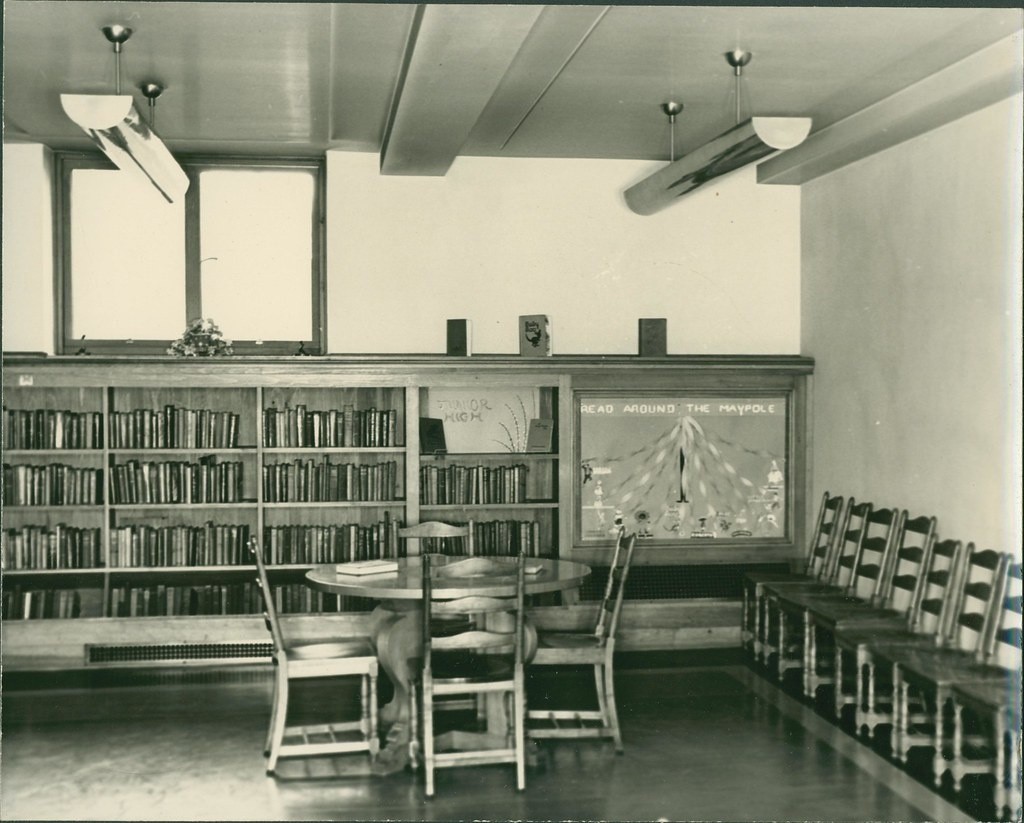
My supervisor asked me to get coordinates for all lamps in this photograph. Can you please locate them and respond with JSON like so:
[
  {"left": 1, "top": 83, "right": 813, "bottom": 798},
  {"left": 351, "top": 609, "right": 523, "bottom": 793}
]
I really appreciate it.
[
  {"left": 623, "top": 52, "right": 812, "bottom": 220},
  {"left": 58, "top": 24, "right": 193, "bottom": 206}
]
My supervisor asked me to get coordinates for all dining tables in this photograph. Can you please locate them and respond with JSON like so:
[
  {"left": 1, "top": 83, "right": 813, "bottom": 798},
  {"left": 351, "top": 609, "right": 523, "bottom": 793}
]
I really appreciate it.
[{"left": 303, "top": 553, "right": 594, "bottom": 770}]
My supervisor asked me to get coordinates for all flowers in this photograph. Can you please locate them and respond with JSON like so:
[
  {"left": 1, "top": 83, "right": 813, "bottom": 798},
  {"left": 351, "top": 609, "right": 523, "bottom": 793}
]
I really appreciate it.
[{"left": 166, "top": 318, "right": 235, "bottom": 359}]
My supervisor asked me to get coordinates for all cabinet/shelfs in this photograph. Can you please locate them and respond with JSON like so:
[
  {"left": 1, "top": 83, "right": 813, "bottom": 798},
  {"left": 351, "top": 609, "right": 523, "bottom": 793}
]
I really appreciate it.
[{"left": 0, "top": 354, "right": 582, "bottom": 688}]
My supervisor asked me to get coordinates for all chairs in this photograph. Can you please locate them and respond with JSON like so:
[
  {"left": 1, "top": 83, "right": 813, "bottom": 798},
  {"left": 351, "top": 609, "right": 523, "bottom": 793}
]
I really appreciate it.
[
  {"left": 396, "top": 516, "right": 487, "bottom": 726},
  {"left": 508, "top": 525, "right": 639, "bottom": 754},
  {"left": 741, "top": 489, "right": 1024, "bottom": 822},
  {"left": 244, "top": 532, "right": 382, "bottom": 780},
  {"left": 406, "top": 548, "right": 528, "bottom": 799}
]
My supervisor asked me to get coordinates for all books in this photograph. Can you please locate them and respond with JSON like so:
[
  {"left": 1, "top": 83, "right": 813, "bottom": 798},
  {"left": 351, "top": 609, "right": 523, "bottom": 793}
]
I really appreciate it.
[{"left": 1, "top": 402, "right": 543, "bottom": 613}]
